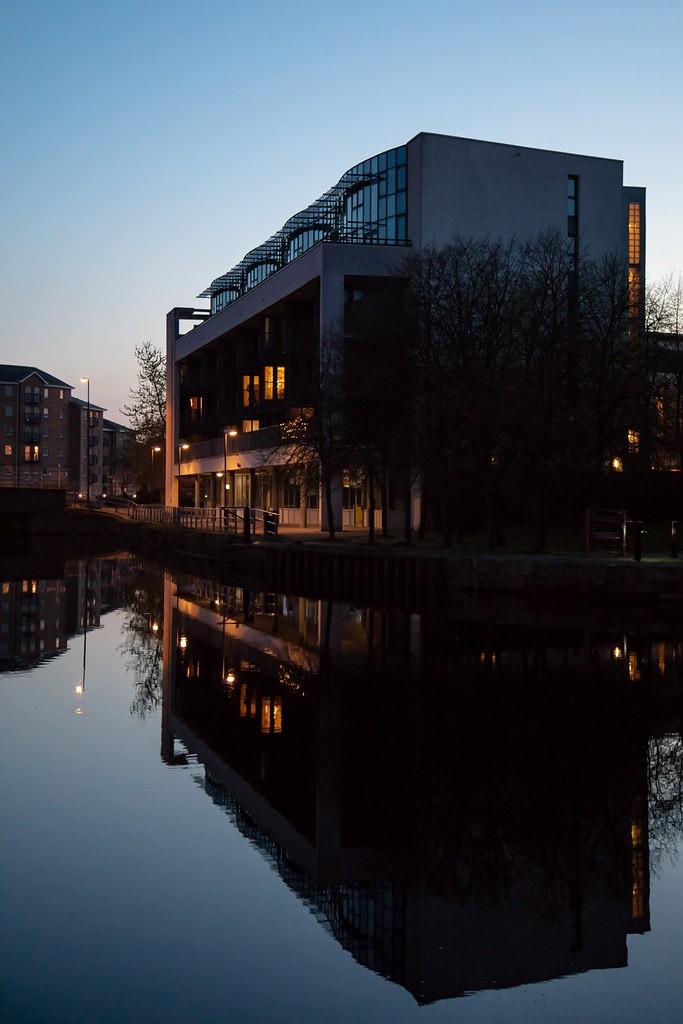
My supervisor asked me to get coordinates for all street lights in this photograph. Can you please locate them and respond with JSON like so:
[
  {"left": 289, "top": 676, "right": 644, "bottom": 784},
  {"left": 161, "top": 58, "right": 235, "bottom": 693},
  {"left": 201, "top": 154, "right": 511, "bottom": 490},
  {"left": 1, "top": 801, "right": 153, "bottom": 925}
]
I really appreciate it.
[
  {"left": 223, "top": 427, "right": 238, "bottom": 533},
  {"left": 220, "top": 605, "right": 235, "bottom": 683},
  {"left": 81, "top": 375, "right": 91, "bottom": 510},
  {"left": 73, "top": 560, "right": 89, "bottom": 695},
  {"left": 149, "top": 444, "right": 163, "bottom": 504},
  {"left": 177, "top": 442, "right": 191, "bottom": 509}
]
